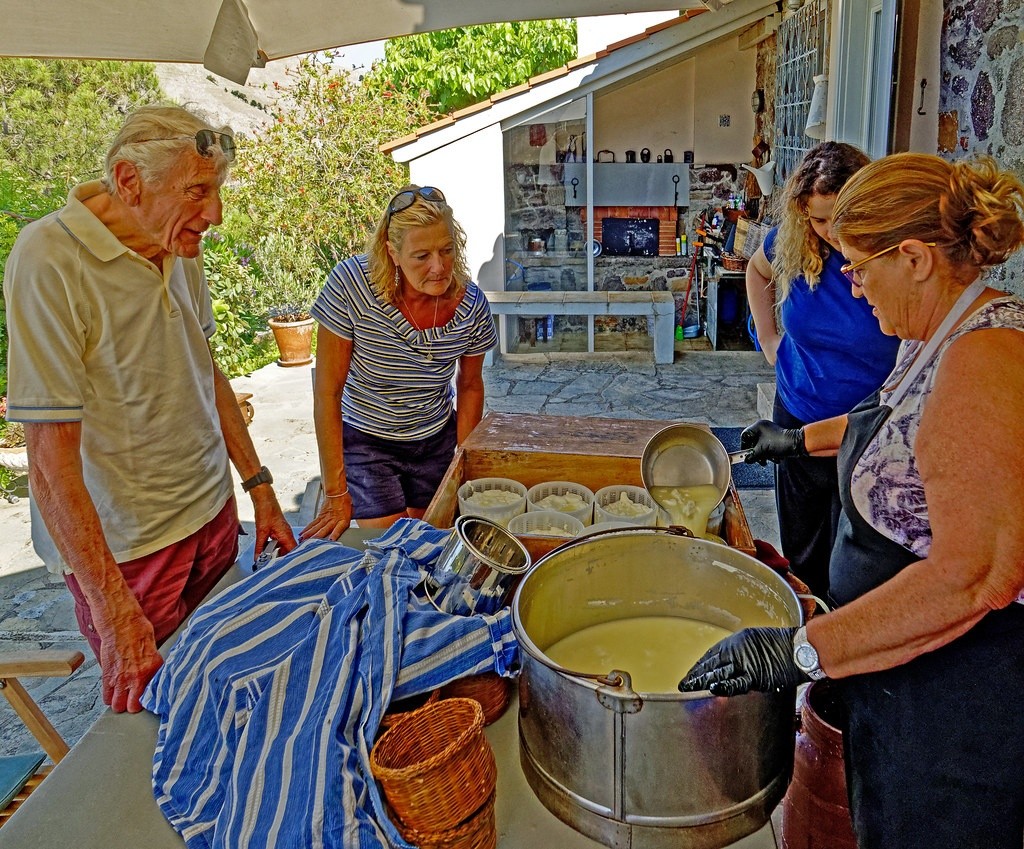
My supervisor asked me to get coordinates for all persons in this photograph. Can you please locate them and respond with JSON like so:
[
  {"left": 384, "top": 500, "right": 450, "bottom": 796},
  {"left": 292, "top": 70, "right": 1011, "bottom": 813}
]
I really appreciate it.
[
  {"left": 3, "top": 101, "right": 297, "bottom": 715},
  {"left": 746, "top": 139, "right": 900, "bottom": 607},
  {"left": 678, "top": 150, "right": 1023, "bottom": 849},
  {"left": 296, "top": 183, "right": 498, "bottom": 542}
]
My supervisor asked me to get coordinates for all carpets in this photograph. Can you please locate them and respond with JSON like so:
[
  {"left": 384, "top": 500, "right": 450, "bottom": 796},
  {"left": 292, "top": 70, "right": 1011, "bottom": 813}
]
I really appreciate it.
[{"left": 709, "top": 426, "right": 777, "bottom": 490}]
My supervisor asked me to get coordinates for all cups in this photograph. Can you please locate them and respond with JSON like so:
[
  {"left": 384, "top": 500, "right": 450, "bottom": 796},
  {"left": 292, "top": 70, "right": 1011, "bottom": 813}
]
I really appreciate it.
[{"left": 530, "top": 238, "right": 542, "bottom": 255}]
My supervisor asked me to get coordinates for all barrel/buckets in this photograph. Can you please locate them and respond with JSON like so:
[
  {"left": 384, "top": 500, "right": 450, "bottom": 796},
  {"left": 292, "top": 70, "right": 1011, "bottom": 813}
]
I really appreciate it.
[
  {"left": 510, "top": 525, "right": 802, "bottom": 849},
  {"left": 527, "top": 481, "right": 594, "bottom": 528},
  {"left": 595, "top": 485, "right": 657, "bottom": 534},
  {"left": 782, "top": 682, "right": 860, "bottom": 849},
  {"left": 657, "top": 502, "right": 728, "bottom": 547},
  {"left": 527, "top": 282, "right": 554, "bottom": 340},
  {"left": 508, "top": 511, "right": 584, "bottom": 537},
  {"left": 577, "top": 521, "right": 655, "bottom": 538},
  {"left": 457, "top": 478, "right": 527, "bottom": 530},
  {"left": 424, "top": 514, "right": 531, "bottom": 616}
]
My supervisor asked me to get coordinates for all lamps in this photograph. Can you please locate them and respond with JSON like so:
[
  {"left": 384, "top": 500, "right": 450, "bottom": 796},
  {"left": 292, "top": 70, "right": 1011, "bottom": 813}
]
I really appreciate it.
[{"left": 750, "top": 89, "right": 762, "bottom": 112}]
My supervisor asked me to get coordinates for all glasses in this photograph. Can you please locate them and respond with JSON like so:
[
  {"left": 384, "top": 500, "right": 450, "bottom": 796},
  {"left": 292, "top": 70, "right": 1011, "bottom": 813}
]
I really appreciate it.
[
  {"left": 134, "top": 129, "right": 237, "bottom": 163},
  {"left": 840, "top": 242, "right": 937, "bottom": 287},
  {"left": 385, "top": 187, "right": 447, "bottom": 230}
]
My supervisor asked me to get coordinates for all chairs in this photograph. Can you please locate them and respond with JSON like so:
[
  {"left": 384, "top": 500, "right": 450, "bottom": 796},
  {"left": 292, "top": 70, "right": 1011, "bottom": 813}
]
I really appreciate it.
[{"left": 0, "top": 650, "right": 85, "bottom": 829}]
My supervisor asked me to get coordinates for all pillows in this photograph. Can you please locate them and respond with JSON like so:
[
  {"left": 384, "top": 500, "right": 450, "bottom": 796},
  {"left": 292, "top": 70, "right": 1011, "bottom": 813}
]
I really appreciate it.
[{"left": 0, "top": 754, "right": 48, "bottom": 813}]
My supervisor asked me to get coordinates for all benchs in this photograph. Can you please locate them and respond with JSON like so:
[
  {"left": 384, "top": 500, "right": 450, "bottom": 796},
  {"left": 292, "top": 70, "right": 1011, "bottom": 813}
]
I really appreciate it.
[{"left": 482, "top": 291, "right": 674, "bottom": 367}]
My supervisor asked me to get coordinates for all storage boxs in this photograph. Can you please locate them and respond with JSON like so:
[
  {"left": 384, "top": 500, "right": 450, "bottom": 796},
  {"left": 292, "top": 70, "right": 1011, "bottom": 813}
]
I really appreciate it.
[{"left": 421, "top": 444, "right": 757, "bottom": 607}]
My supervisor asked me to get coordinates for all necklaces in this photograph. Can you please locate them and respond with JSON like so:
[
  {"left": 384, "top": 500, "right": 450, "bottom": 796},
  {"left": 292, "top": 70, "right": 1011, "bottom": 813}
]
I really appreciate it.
[{"left": 401, "top": 292, "right": 439, "bottom": 362}]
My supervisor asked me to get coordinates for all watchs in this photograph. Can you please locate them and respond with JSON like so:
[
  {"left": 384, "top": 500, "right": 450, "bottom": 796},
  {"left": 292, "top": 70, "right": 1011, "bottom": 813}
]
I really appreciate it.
[
  {"left": 792, "top": 625, "right": 828, "bottom": 682},
  {"left": 241, "top": 465, "right": 274, "bottom": 493}
]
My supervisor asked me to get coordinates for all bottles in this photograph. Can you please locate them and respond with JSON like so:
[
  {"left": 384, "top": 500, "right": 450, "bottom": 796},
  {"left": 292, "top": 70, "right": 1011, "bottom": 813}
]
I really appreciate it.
[
  {"left": 681, "top": 235, "right": 686, "bottom": 255},
  {"left": 722, "top": 194, "right": 745, "bottom": 210},
  {"left": 677, "top": 235, "right": 680, "bottom": 256}
]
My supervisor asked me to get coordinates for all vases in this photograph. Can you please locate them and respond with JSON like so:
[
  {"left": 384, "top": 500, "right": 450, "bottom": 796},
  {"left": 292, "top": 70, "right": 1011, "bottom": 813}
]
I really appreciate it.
[
  {"left": 267, "top": 317, "right": 316, "bottom": 367},
  {"left": 0, "top": 438, "right": 30, "bottom": 475}
]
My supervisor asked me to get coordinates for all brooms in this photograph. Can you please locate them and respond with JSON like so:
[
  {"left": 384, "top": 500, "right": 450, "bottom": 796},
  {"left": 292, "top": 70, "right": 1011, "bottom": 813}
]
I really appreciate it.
[{"left": 675, "top": 208, "right": 707, "bottom": 341}]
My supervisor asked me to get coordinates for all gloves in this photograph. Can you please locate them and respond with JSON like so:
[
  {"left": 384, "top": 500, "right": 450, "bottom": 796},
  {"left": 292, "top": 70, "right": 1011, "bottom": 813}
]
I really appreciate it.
[
  {"left": 677, "top": 626, "right": 814, "bottom": 697},
  {"left": 740, "top": 419, "right": 811, "bottom": 466}
]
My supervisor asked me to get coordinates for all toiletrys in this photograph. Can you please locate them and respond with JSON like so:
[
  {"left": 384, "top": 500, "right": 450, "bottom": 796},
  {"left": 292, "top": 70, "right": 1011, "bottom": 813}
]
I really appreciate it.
[{"left": 727, "top": 191, "right": 743, "bottom": 209}]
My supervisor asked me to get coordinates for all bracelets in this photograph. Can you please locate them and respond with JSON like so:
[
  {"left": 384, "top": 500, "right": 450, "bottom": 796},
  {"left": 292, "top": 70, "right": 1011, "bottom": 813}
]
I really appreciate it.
[{"left": 323, "top": 487, "right": 349, "bottom": 498}]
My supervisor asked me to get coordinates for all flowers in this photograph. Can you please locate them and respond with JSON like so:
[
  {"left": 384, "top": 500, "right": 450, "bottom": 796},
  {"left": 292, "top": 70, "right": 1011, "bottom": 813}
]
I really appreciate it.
[{"left": 0, "top": 396, "right": 28, "bottom": 449}]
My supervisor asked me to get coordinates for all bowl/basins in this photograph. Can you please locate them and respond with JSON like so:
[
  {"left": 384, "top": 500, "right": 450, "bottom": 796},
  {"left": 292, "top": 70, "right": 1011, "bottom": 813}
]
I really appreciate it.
[
  {"left": 722, "top": 207, "right": 750, "bottom": 223},
  {"left": 752, "top": 140, "right": 770, "bottom": 159}
]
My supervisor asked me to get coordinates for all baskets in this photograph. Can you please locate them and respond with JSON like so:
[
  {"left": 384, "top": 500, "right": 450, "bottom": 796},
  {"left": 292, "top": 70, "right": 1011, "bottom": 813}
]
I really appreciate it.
[
  {"left": 368, "top": 696, "right": 499, "bottom": 834},
  {"left": 385, "top": 784, "right": 497, "bottom": 848},
  {"left": 381, "top": 687, "right": 442, "bottom": 731},
  {"left": 442, "top": 672, "right": 510, "bottom": 729}
]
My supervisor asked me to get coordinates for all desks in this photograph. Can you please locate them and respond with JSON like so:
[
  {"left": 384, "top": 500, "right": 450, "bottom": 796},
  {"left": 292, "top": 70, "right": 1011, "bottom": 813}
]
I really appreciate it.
[
  {"left": 463, "top": 409, "right": 709, "bottom": 457},
  {"left": 0, "top": 523, "right": 775, "bottom": 849},
  {"left": 707, "top": 257, "right": 748, "bottom": 350}
]
